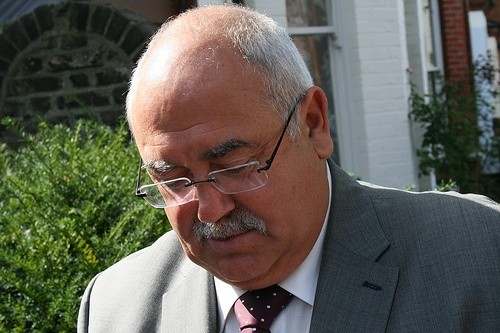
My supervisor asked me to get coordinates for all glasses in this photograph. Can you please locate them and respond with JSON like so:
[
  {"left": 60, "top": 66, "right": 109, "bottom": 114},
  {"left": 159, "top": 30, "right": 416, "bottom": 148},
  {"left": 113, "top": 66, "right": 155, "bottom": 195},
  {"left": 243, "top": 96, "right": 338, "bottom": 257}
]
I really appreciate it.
[{"left": 135, "top": 98, "right": 303, "bottom": 208}]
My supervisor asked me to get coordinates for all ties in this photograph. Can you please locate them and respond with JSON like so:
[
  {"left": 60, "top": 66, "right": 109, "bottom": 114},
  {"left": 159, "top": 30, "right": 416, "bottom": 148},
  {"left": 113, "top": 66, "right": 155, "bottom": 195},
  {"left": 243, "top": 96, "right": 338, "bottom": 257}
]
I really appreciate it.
[{"left": 232, "top": 285, "right": 296, "bottom": 333}]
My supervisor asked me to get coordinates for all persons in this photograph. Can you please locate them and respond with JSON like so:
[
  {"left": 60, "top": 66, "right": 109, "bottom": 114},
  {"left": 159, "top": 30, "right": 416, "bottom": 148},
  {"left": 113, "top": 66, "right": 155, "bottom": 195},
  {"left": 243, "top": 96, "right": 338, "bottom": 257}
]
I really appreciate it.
[{"left": 77, "top": 5, "right": 500, "bottom": 333}]
